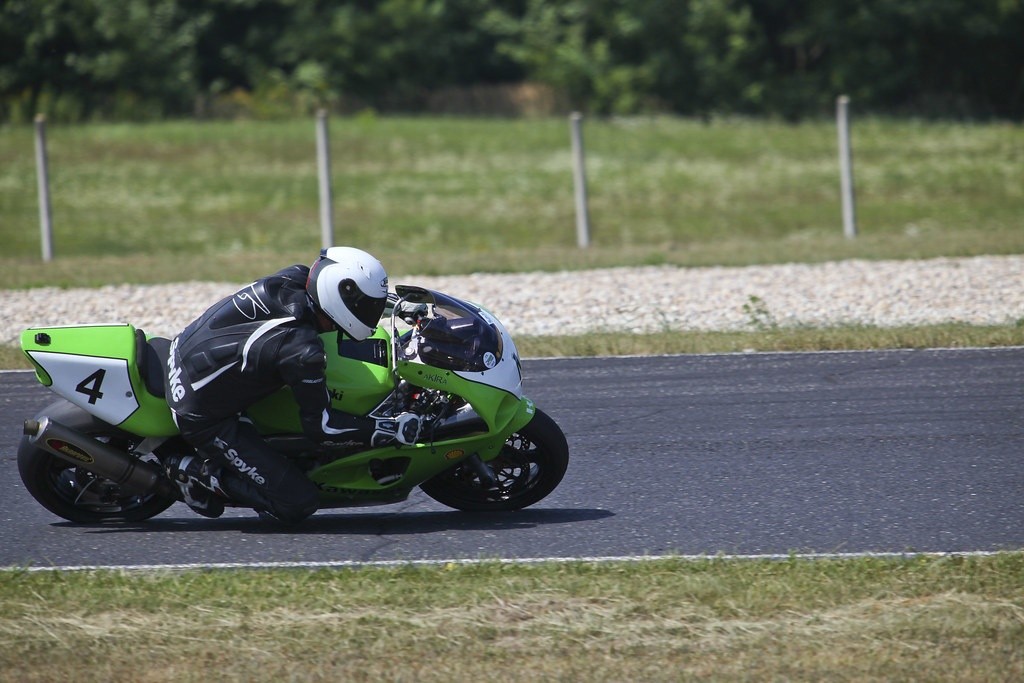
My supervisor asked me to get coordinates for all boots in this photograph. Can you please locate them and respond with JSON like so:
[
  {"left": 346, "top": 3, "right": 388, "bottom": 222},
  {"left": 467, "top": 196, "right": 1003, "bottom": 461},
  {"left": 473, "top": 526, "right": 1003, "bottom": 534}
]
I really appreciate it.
[{"left": 164, "top": 452, "right": 225, "bottom": 518}]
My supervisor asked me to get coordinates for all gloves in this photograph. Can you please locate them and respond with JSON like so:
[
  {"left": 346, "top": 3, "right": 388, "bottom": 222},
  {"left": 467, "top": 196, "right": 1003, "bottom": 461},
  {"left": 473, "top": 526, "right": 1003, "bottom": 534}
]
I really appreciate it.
[
  {"left": 380, "top": 292, "right": 429, "bottom": 325},
  {"left": 371, "top": 412, "right": 424, "bottom": 449}
]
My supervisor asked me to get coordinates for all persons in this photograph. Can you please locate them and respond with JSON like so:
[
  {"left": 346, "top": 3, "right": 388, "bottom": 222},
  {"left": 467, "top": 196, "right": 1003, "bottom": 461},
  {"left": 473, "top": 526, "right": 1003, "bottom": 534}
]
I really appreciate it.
[{"left": 165, "top": 247, "right": 435, "bottom": 528}]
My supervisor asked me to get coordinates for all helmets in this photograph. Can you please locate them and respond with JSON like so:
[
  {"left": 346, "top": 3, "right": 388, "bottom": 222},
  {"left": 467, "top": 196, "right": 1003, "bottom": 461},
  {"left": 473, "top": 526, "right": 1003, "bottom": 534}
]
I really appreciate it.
[{"left": 306, "top": 246, "right": 388, "bottom": 342}]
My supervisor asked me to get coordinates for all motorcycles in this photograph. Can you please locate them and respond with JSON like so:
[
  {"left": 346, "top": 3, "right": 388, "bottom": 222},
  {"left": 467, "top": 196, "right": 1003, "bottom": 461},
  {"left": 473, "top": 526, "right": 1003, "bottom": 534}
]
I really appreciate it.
[{"left": 13, "top": 281, "right": 571, "bottom": 528}]
